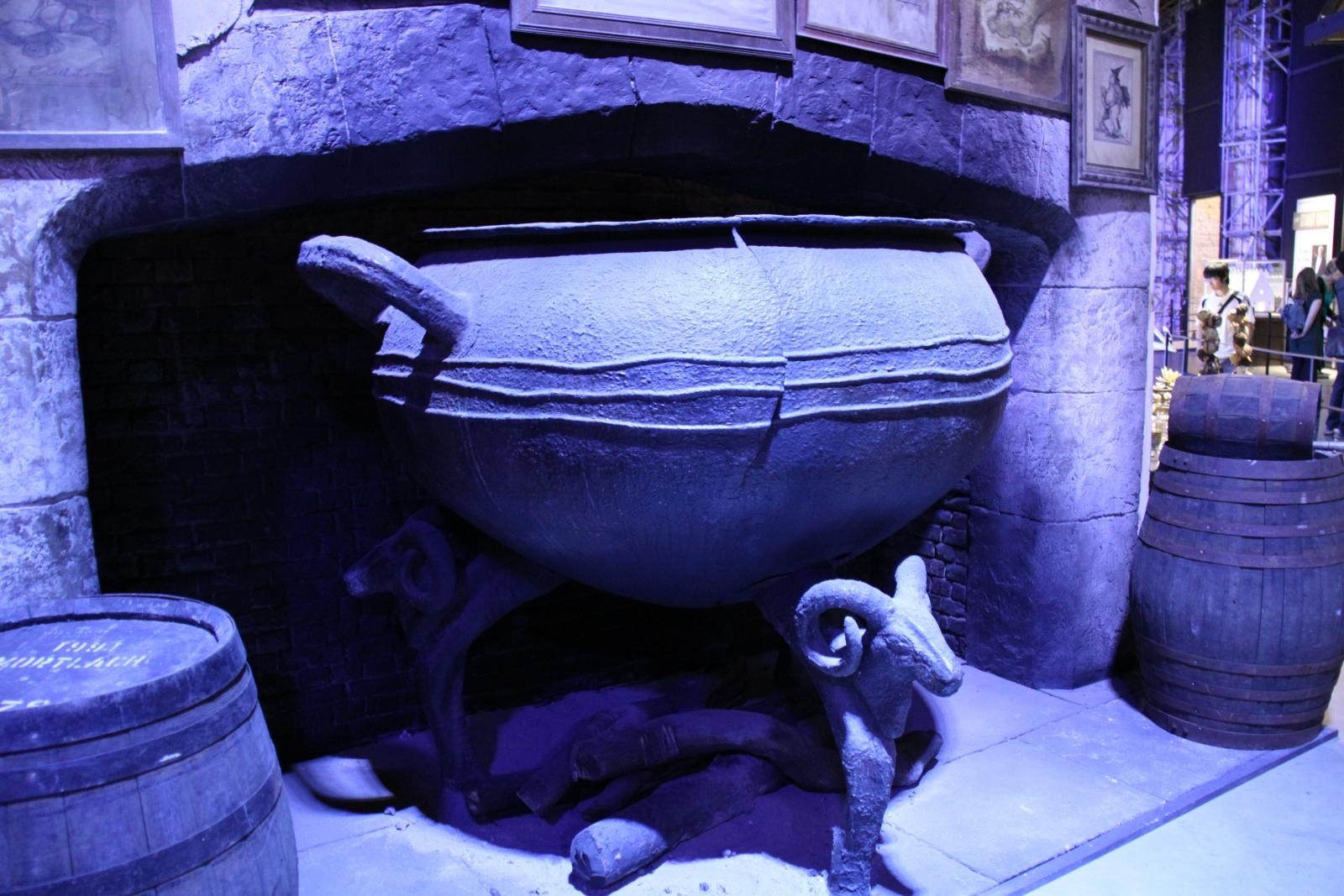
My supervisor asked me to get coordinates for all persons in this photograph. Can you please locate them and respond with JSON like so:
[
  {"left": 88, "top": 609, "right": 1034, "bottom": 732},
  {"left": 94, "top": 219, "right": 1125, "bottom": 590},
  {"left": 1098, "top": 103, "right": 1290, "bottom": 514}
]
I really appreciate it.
[
  {"left": 1289, "top": 268, "right": 1324, "bottom": 382},
  {"left": 1317, "top": 258, "right": 1338, "bottom": 378},
  {"left": 1324, "top": 251, "right": 1343, "bottom": 439},
  {"left": 1195, "top": 261, "right": 1256, "bottom": 376}
]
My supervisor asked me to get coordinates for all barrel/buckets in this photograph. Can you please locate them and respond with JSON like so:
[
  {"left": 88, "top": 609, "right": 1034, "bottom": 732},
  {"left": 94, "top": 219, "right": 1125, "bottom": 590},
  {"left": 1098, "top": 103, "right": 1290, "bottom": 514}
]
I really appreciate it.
[
  {"left": 1128, "top": 446, "right": 1340, "bottom": 749},
  {"left": 1167, "top": 374, "right": 1322, "bottom": 454},
  {"left": 0, "top": 590, "right": 303, "bottom": 896}
]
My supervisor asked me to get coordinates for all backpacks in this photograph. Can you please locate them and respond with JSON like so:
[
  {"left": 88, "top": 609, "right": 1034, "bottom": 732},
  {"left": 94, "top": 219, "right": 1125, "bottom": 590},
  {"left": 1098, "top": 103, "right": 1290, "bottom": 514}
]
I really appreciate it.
[{"left": 1282, "top": 296, "right": 1307, "bottom": 334}]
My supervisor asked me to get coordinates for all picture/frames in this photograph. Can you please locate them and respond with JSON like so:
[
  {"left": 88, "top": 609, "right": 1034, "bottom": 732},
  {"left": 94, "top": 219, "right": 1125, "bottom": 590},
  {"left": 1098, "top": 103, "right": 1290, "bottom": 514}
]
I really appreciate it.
[
  {"left": 945, "top": 1, "right": 1076, "bottom": 118},
  {"left": 1, "top": 0, "right": 184, "bottom": 156},
  {"left": 507, "top": 0, "right": 799, "bottom": 68},
  {"left": 1066, "top": 4, "right": 1160, "bottom": 197},
  {"left": 790, "top": 0, "right": 945, "bottom": 75}
]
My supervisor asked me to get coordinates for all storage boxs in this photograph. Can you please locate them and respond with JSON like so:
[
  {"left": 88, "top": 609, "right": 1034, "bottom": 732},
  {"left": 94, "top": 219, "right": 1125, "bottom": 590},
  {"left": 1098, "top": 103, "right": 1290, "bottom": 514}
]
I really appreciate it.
[{"left": 1219, "top": 257, "right": 1286, "bottom": 314}]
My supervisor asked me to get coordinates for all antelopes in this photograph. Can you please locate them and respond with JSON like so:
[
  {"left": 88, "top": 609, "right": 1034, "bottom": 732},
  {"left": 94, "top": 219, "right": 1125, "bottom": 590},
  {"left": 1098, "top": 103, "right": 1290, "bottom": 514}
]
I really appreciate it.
[{"left": 792, "top": 554, "right": 964, "bottom": 738}]
